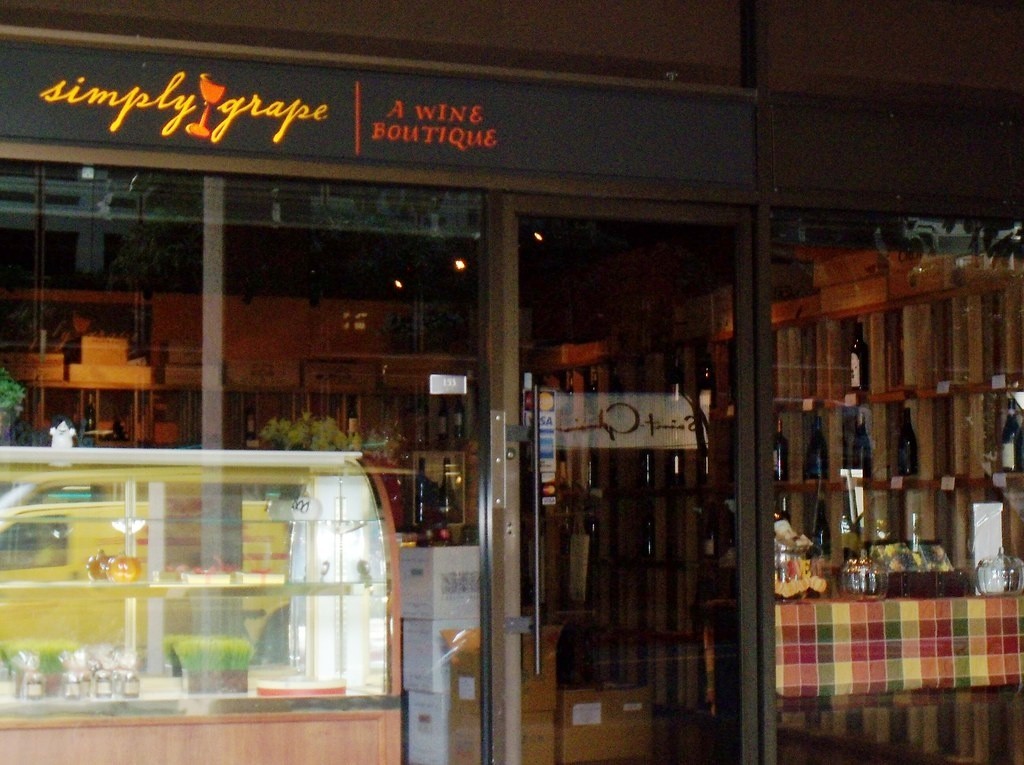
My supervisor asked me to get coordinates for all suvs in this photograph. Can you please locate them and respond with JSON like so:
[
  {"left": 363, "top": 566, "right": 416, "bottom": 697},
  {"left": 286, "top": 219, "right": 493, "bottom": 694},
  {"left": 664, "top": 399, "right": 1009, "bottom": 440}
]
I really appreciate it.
[
  {"left": 0, "top": 502, "right": 295, "bottom": 663},
  {"left": 0, "top": 456, "right": 399, "bottom": 678}
]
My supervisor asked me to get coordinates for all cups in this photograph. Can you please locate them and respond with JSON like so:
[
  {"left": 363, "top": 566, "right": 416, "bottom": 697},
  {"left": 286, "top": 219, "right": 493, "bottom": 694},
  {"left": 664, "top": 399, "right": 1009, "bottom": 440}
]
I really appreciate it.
[
  {"left": 976, "top": 547, "right": 1024, "bottom": 596},
  {"left": 836, "top": 549, "right": 889, "bottom": 601}
]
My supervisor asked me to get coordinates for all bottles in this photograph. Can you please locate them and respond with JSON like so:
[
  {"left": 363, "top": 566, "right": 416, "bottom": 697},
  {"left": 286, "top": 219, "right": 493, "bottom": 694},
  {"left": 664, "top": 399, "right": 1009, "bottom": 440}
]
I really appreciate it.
[
  {"left": 437, "top": 458, "right": 456, "bottom": 523},
  {"left": 588, "top": 372, "right": 598, "bottom": 392},
  {"left": 245, "top": 403, "right": 256, "bottom": 441},
  {"left": 1000, "top": 399, "right": 1024, "bottom": 472},
  {"left": 807, "top": 416, "right": 827, "bottom": 479},
  {"left": 779, "top": 498, "right": 791, "bottom": 525},
  {"left": 897, "top": 407, "right": 917, "bottom": 475},
  {"left": 452, "top": 394, "right": 463, "bottom": 438},
  {"left": 437, "top": 397, "right": 447, "bottom": 437},
  {"left": 704, "top": 509, "right": 718, "bottom": 558},
  {"left": 850, "top": 322, "right": 869, "bottom": 391},
  {"left": 415, "top": 458, "right": 432, "bottom": 523},
  {"left": 851, "top": 413, "right": 871, "bottom": 477},
  {"left": 348, "top": 396, "right": 359, "bottom": 433},
  {"left": 566, "top": 378, "right": 574, "bottom": 395},
  {"left": 771, "top": 420, "right": 788, "bottom": 479},
  {"left": 816, "top": 530, "right": 827, "bottom": 557},
  {"left": 84, "top": 394, "right": 96, "bottom": 432}
]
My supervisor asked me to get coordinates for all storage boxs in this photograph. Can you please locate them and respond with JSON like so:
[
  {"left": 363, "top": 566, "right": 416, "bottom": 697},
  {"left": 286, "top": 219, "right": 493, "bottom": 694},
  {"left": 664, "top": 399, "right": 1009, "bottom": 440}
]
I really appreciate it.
[
  {"left": 0, "top": 334, "right": 473, "bottom": 392},
  {"left": 534, "top": 287, "right": 737, "bottom": 368},
  {"left": 400, "top": 542, "right": 654, "bottom": 765},
  {"left": 770, "top": 244, "right": 1024, "bottom": 318}
]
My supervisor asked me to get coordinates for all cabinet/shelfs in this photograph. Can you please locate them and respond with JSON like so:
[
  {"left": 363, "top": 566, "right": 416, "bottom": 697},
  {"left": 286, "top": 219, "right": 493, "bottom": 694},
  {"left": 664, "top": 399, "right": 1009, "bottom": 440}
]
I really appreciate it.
[
  {"left": 771, "top": 276, "right": 1024, "bottom": 765},
  {"left": 0, "top": 388, "right": 482, "bottom": 547},
  {"left": 0, "top": 444, "right": 402, "bottom": 765},
  {"left": 528, "top": 332, "right": 738, "bottom": 718}
]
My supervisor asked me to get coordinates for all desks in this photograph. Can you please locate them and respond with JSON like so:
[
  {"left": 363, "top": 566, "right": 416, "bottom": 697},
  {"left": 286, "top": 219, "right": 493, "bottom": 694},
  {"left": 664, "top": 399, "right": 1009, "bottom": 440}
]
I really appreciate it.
[{"left": 697, "top": 594, "right": 1024, "bottom": 765}]
